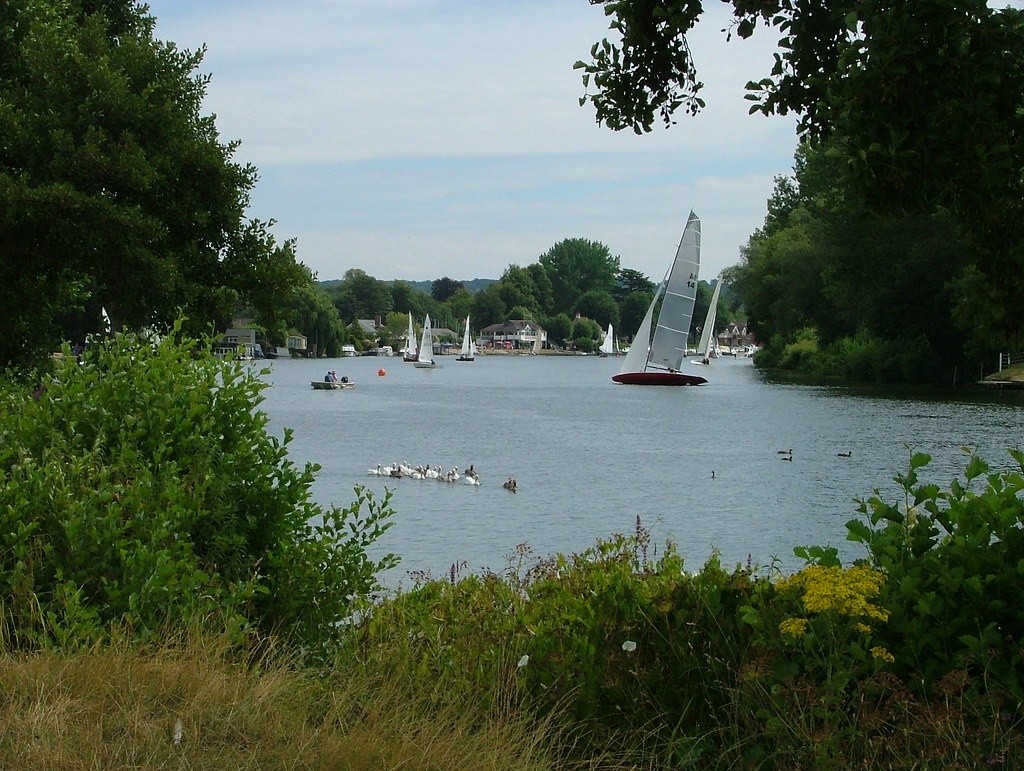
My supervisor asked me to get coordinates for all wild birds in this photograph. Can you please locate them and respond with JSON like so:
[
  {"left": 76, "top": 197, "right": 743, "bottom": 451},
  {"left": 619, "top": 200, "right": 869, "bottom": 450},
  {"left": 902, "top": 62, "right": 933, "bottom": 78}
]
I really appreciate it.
[
  {"left": 710, "top": 471, "right": 715, "bottom": 478},
  {"left": 838, "top": 451, "right": 852, "bottom": 457},
  {"left": 367, "top": 462, "right": 479, "bottom": 485},
  {"left": 778, "top": 449, "right": 792, "bottom": 461},
  {"left": 503, "top": 478, "right": 517, "bottom": 489}
]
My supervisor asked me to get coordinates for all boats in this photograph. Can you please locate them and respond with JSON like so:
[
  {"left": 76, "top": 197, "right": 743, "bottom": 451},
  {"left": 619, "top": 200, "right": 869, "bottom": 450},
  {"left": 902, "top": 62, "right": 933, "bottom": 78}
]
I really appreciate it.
[{"left": 311, "top": 381, "right": 357, "bottom": 390}]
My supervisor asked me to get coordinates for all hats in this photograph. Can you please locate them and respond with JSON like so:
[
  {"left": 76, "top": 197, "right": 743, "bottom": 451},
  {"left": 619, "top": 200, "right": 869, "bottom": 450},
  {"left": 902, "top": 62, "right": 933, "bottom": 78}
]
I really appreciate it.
[
  {"left": 328, "top": 372, "right": 331, "bottom": 374},
  {"left": 332, "top": 371, "right": 336, "bottom": 374}
]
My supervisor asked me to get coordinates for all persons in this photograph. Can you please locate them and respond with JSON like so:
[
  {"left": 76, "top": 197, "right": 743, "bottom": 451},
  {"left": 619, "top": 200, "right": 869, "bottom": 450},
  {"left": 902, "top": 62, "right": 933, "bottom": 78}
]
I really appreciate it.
[
  {"left": 325, "top": 370, "right": 337, "bottom": 382},
  {"left": 702, "top": 359, "right": 709, "bottom": 365}
]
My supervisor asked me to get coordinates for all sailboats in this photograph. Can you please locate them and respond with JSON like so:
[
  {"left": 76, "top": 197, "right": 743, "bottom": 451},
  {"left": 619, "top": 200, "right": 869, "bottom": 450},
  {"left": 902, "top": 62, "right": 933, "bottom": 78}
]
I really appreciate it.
[
  {"left": 414, "top": 313, "right": 436, "bottom": 368},
  {"left": 456, "top": 315, "right": 474, "bottom": 362},
  {"left": 597, "top": 322, "right": 614, "bottom": 359},
  {"left": 402, "top": 309, "right": 419, "bottom": 362},
  {"left": 530, "top": 329, "right": 542, "bottom": 356},
  {"left": 613, "top": 209, "right": 709, "bottom": 385},
  {"left": 683, "top": 271, "right": 760, "bottom": 365}
]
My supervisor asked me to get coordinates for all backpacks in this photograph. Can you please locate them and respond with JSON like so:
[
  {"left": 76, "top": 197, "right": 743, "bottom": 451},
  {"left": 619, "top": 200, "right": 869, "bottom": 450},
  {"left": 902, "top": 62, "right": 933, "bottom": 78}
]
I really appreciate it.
[{"left": 325, "top": 375, "right": 331, "bottom": 382}]
